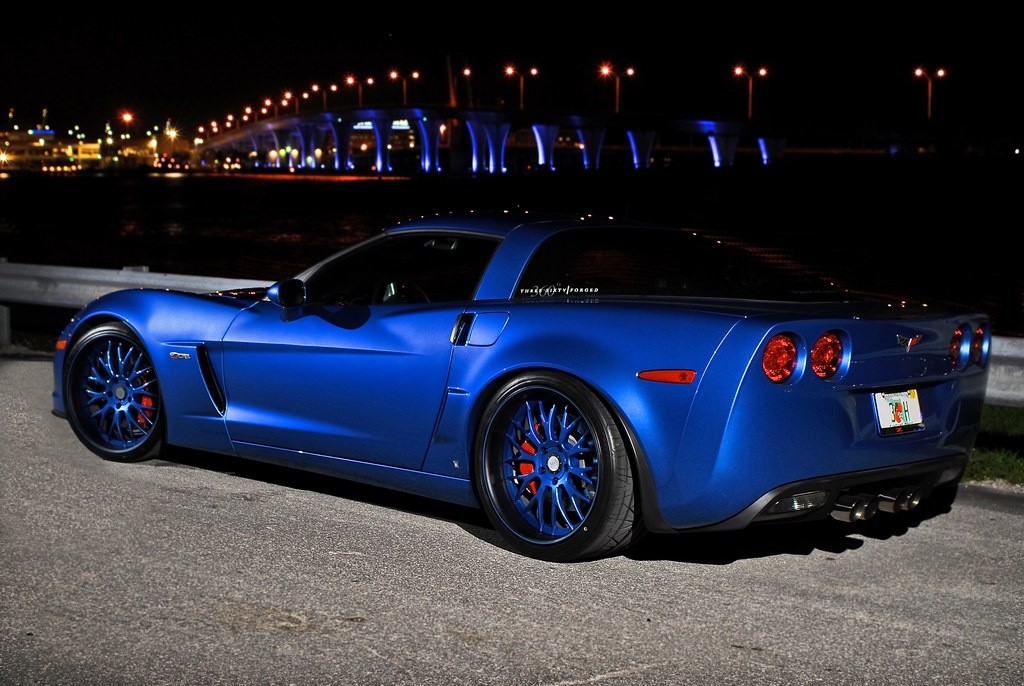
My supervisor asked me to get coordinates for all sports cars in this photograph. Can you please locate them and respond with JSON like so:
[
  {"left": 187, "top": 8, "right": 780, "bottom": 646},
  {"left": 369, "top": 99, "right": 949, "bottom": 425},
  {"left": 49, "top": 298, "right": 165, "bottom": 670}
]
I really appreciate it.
[{"left": 47, "top": 208, "right": 993, "bottom": 561}]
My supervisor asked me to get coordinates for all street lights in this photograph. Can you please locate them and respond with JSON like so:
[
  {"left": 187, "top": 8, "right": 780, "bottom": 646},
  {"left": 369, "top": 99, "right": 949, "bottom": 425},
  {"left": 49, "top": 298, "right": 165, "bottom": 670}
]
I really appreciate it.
[
  {"left": 506, "top": 66, "right": 539, "bottom": 115},
  {"left": 913, "top": 68, "right": 946, "bottom": 120},
  {"left": 601, "top": 65, "right": 635, "bottom": 115},
  {"left": 346, "top": 76, "right": 373, "bottom": 109},
  {"left": 734, "top": 66, "right": 767, "bottom": 117},
  {"left": 390, "top": 70, "right": 420, "bottom": 104}
]
